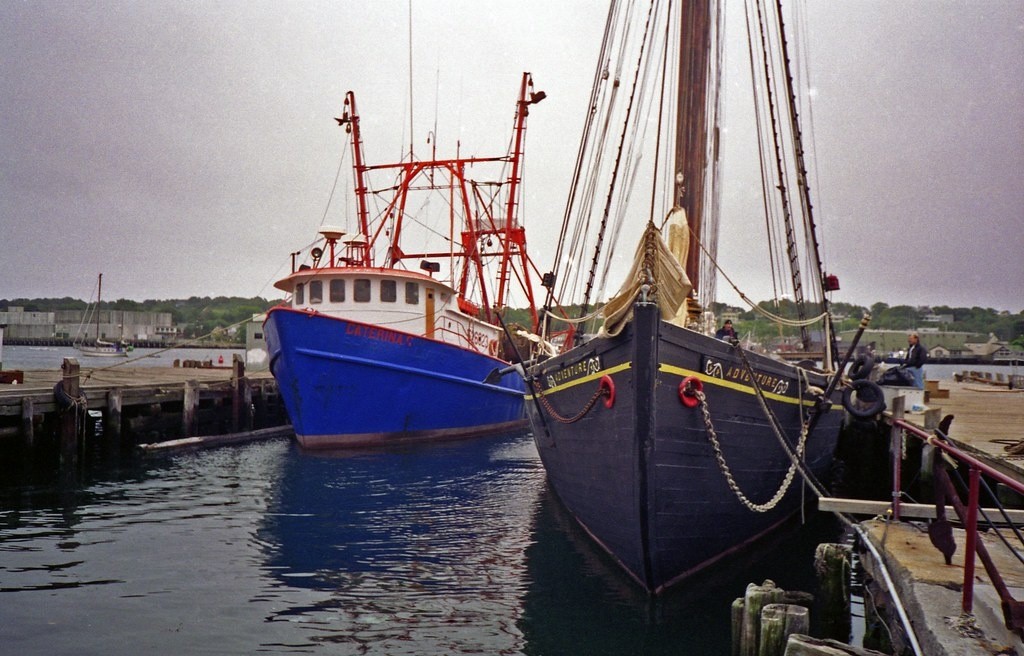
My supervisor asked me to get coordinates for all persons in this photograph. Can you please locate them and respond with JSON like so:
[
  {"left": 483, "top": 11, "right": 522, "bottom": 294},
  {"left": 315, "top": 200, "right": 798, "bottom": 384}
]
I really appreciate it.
[
  {"left": 716, "top": 319, "right": 738, "bottom": 345},
  {"left": 904, "top": 333, "right": 927, "bottom": 411}
]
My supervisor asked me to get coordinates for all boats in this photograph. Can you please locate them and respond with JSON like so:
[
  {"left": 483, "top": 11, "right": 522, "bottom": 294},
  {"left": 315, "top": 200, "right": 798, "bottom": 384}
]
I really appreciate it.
[
  {"left": 262, "top": 70, "right": 555, "bottom": 452},
  {"left": 277, "top": 424, "right": 532, "bottom": 591},
  {"left": 774, "top": 351, "right": 847, "bottom": 361}
]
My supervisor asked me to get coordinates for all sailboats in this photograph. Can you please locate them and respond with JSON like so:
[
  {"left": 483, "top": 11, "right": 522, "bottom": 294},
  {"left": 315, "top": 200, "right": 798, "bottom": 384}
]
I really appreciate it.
[
  {"left": 73, "top": 273, "right": 129, "bottom": 358},
  {"left": 521, "top": 0, "right": 867, "bottom": 645}
]
